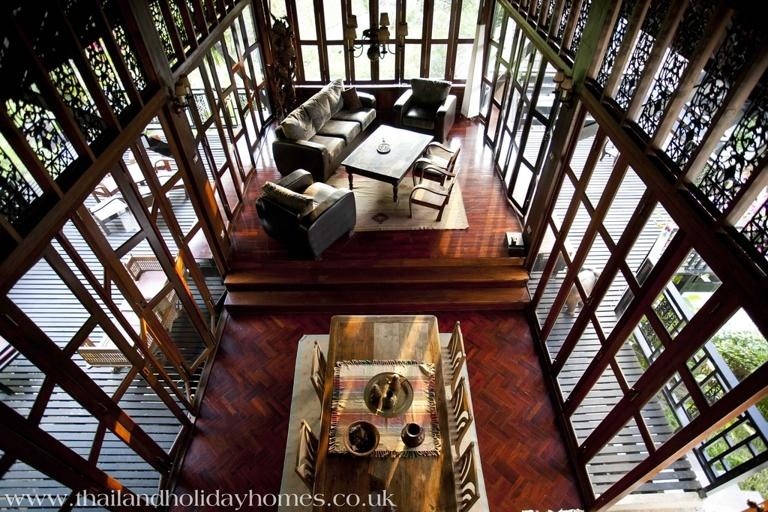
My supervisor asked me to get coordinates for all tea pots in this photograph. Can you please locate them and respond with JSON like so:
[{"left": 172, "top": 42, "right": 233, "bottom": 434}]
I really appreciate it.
[{"left": 385, "top": 374, "right": 408, "bottom": 394}]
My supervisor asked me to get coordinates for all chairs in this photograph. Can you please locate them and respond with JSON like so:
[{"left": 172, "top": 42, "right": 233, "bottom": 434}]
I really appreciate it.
[
  {"left": 444, "top": 320, "right": 467, "bottom": 387},
  {"left": 256, "top": 168, "right": 356, "bottom": 261},
  {"left": 450, "top": 377, "right": 472, "bottom": 449},
  {"left": 310, "top": 340, "right": 327, "bottom": 402},
  {"left": 453, "top": 442, "right": 481, "bottom": 512},
  {"left": 411, "top": 142, "right": 461, "bottom": 187},
  {"left": 394, "top": 79, "right": 457, "bottom": 142},
  {"left": 294, "top": 418, "right": 316, "bottom": 491},
  {"left": 408, "top": 166, "right": 461, "bottom": 222},
  {"left": 88, "top": 135, "right": 190, "bottom": 232}
]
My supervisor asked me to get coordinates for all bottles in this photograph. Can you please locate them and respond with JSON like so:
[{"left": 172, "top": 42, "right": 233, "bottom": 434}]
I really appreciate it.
[{"left": 382, "top": 391, "right": 396, "bottom": 409}]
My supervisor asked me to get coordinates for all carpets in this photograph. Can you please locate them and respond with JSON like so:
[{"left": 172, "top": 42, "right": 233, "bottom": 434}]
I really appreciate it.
[
  {"left": 327, "top": 144, "right": 468, "bottom": 233},
  {"left": 279, "top": 334, "right": 490, "bottom": 512}
]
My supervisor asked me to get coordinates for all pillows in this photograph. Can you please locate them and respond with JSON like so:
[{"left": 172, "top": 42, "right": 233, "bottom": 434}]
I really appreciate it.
[
  {"left": 279, "top": 79, "right": 363, "bottom": 142},
  {"left": 411, "top": 78, "right": 454, "bottom": 104},
  {"left": 261, "top": 180, "right": 313, "bottom": 214}
]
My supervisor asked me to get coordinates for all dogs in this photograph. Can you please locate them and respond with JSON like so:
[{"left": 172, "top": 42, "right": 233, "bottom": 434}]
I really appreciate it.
[{"left": 565, "top": 268, "right": 600, "bottom": 316}]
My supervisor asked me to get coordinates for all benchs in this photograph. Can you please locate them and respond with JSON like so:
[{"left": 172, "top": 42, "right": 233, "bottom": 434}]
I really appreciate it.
[{"left": 75, "top": 256, "right": 193, "bottom": 379}]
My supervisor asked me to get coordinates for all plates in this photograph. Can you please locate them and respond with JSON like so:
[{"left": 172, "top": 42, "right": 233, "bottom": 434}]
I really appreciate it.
[{"left": 364, "top": 370, "right": 413, "bottom": 417}]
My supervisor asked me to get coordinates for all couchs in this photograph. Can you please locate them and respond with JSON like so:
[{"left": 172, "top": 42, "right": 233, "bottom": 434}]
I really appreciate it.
[{"left": 272, "top": 81, "right": 375, "bottom": 183}]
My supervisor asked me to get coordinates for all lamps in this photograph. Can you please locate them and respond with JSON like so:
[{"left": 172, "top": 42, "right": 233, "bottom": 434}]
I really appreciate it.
[
  {"left": 343, "top": 0, "right": 408, "bottom": 61},
  {"left": 552, "top": 70, "right": 572, "bottom": 103},
  {"left": 172, "top": 75, "right": 192, "bottom": 113}
]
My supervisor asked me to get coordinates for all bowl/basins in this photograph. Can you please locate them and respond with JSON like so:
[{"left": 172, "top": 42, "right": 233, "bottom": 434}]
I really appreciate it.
[{"left": 343, "top": 420, "right": 380, "bottom": 456}]
